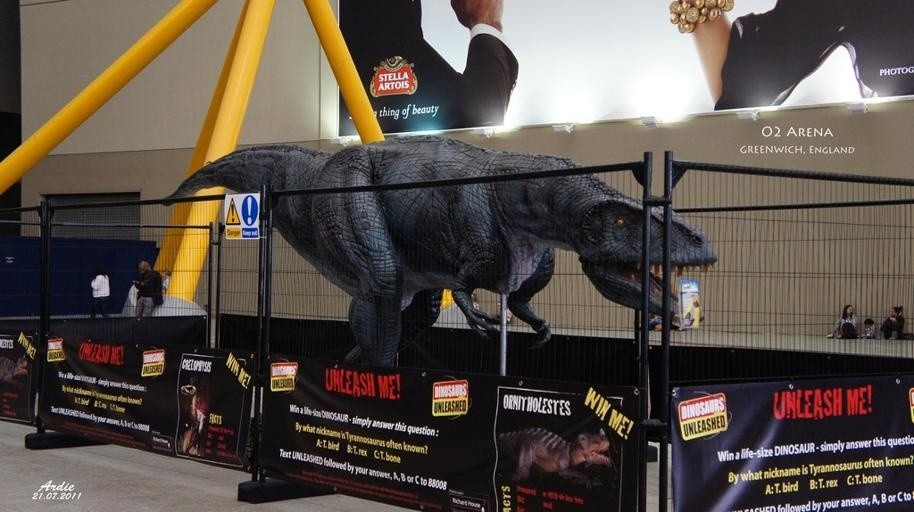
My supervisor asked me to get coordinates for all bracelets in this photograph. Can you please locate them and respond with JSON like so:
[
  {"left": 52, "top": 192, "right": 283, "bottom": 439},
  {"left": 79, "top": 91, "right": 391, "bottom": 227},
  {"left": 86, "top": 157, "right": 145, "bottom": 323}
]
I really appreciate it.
[{"left": 669, "top": 0, "right": 734, "bottom": 36}]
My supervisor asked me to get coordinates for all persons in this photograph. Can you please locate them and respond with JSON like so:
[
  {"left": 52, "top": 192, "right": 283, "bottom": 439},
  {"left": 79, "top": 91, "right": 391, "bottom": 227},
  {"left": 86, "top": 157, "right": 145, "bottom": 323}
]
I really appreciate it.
[
  {"left": 879, "top": 306, "right": 906, "bottom": 339},
  {"left": 826, "top": 305, "right": 856, "bottom": 339},
  {"left": 338, "top": 1, "right": 519, "bottom": 134},
  {"left": 87, "top": 263, "right": 113, "bottom": 319},
  {"left": 840, "top": 322, "right": 858, "bottom": 339},
  {"left": 669, "top": 0, "right": 914, "bottom": 113},
  {"left": 861, "top": 318, "right": 877, "bottom": 338},
  {"left": 132, "top": 258, "right": 164, "bottom": 318}
]
